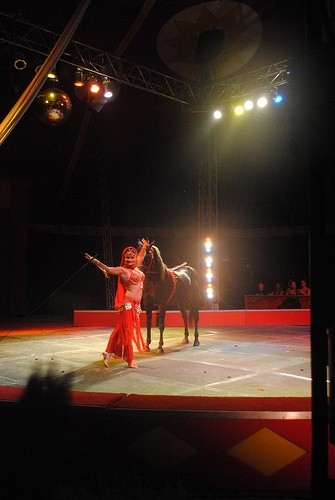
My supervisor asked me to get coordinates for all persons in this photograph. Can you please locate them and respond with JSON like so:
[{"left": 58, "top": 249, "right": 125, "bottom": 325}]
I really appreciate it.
[
  {"left": 85, "top": 239, "right": 149, "bottom": 369},
  {"left": 256, "top": 280, "right": 310, "bottom": 295}
]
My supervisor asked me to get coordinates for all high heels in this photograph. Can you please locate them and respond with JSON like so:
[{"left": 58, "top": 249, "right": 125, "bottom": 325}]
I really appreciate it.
[
  {"left": 123, "top": 356, "right": 137, "bottom": 367},
  {"left": 100, "top": 352, "right": 110, "bottom": 368}
]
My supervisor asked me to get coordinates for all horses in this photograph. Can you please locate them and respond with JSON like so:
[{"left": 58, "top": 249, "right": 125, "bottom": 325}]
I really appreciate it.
[{"left": 138, "top": 239, "right": 202, "bottom": 356}]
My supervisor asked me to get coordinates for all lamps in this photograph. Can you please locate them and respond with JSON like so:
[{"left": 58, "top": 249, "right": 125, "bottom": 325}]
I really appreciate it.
[{"left": 72, "top": 66, "right": 114, "bottom": 99}]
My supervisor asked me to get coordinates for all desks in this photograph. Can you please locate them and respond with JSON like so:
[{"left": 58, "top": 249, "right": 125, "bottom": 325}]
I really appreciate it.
[{"left": 244, "top": 294, "right": 311, "bottom": 309}]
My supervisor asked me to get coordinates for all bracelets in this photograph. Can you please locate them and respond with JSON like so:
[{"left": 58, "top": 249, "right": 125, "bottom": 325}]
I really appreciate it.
[{"left": 97, "top": 261, "right": 101, "bottom": 266}]
